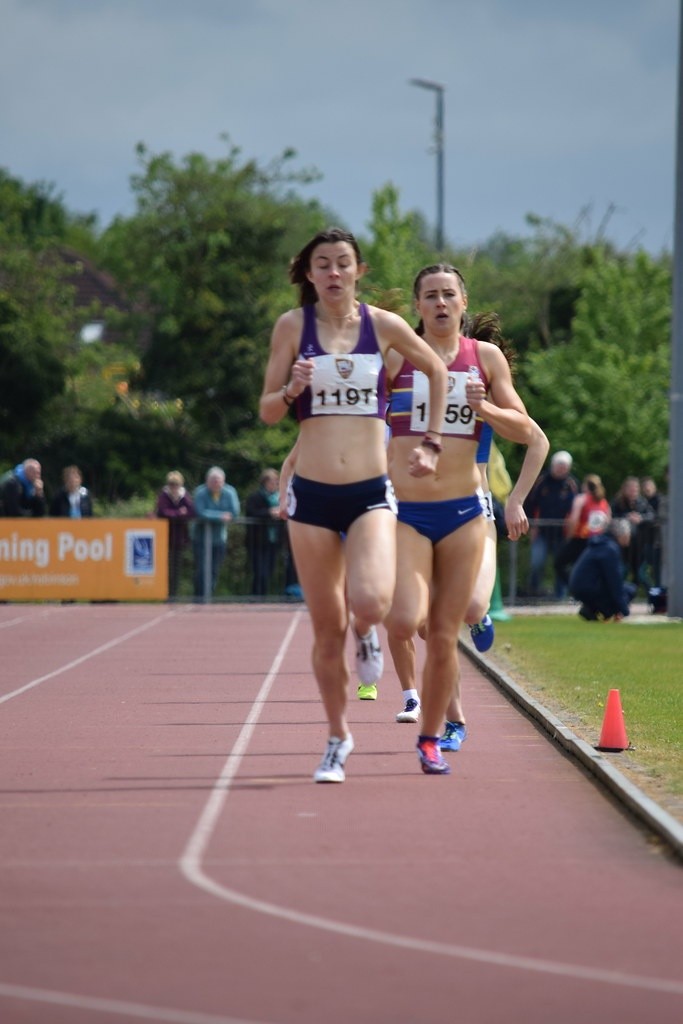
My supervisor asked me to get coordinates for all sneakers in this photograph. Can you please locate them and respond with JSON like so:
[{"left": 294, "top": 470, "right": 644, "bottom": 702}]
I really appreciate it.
[
  {"left": 314, "top": 730, "right": 354, "bottom": 782},
  {"left": 416, "top": 740, "right": 450, "bottom": 774},
  {"left": 396, "top": 698, "right": 421, "bottom": 722},
  {"left": 357, "top": 681, "right": 377, "bottom": 699},
  {"left": 470, "top": 613, "right": 494, "bottom": 652},
  {"left": 439, "top": 720, "right": 465, "bottom": 751},
  {"left": 351, "top": 616, "right": 384, "bottom": 686}
]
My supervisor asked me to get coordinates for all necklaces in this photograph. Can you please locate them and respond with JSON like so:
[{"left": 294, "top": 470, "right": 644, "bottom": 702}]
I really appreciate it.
[{"left": 321, "top": 306, "right": 357, "bottom": 318}]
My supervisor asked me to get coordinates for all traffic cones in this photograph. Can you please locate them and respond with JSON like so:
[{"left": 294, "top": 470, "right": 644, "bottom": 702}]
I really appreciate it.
[
  {"left": 486, "top": 568, "right": 511, "bottom": 622},
  {"left": 592, "top": 688, "right": 636, "bottom": 753}
]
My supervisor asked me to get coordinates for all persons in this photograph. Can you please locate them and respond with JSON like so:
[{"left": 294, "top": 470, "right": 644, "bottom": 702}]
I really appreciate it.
[
  {"left": 275, "top": 431, "right": 379, "bottom": 700},
  {"left": 382, "top": 616, "right": 432, "bottom": 726},
  {"left": 377, "top": 263, "right": 531, "bottom": 777},
  {"left": 462, "top": 310, "right": 550, "bottom": 654},
  {"left": 258, "top": 227, "right": 448, "bottom": 784},
  {"left": 1, "top": 449, "right": 667, "bottom": 624}
]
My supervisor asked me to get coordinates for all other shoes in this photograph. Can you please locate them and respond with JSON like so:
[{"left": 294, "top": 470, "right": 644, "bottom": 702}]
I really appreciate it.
[{"left": 579, "top": 610, "right": 598, "bottom": 621}]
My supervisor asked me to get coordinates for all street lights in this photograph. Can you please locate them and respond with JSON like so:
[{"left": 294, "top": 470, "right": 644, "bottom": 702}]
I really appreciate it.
[{"left": 406, "top": 77, "right": 447, "bottom": 250}]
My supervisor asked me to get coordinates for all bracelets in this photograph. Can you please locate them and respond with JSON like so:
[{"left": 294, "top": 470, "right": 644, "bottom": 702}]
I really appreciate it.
[
  {"left": 423, "top": 430, "right": 441, "bottom": 453},
  {"left": 282, "top": 385, "right": 297, "bottom": 406}
]
